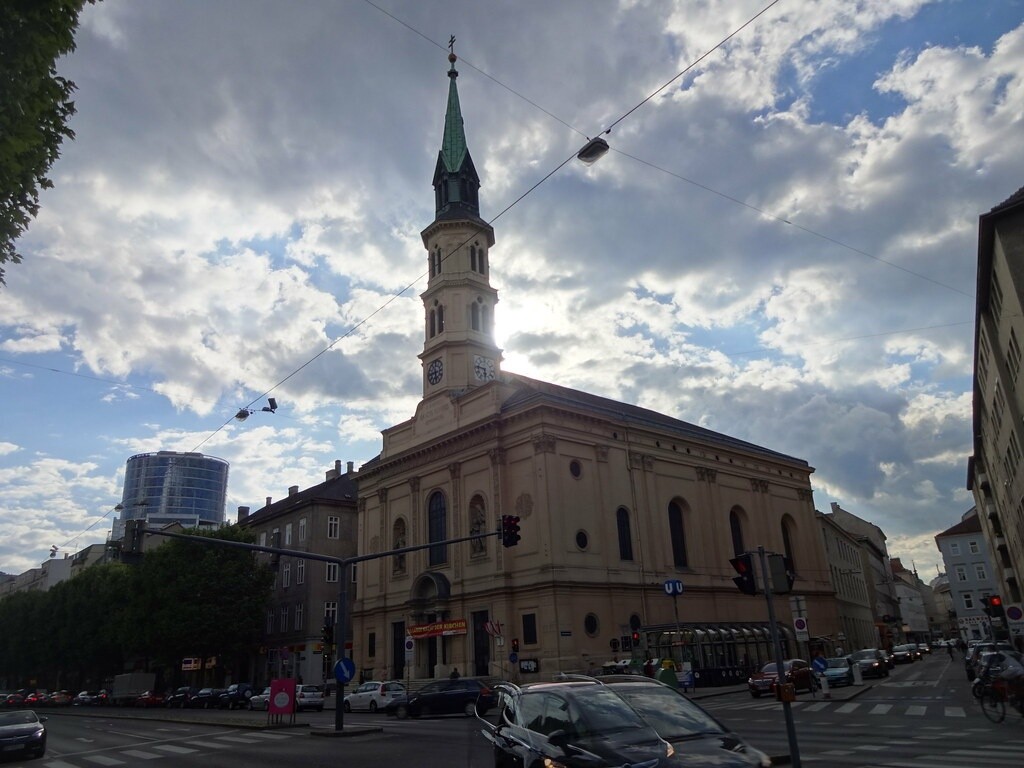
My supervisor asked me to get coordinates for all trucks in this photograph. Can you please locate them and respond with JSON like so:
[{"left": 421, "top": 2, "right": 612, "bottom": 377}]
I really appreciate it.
[{"left": 108, "top": 671, "right": 156, "bottom": 706}]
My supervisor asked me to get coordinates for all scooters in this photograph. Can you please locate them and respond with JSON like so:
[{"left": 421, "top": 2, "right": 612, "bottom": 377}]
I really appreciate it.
[{"left": 970, "top": 650, "right": 1024, "bottom": 722}]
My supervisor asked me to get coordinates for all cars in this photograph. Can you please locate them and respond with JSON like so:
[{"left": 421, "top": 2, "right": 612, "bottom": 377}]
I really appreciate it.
[
  {"left": 823, "top": 656, "right": 855, "bottom": 687},
  {"left": 747, "top": 657, "right": 820, "bottom": 701},
  {"left": 247, "top": 686, "right": 271, "bottom": 710},
  {"left": 296, "top": 683, "right": 325, "bottom": 710},
  {"left": 964, "top": 639, "right": 1023, "bottom": 693},
  {"left": 165, "top": 685, "right": 199, "bottom": 709},
  {"left": 0, "top": 709, "right": 48, "bottom": 764},
  {"left": 880, "top": 649, "right": 896, "bottom": 669},
  {"left": 384, "top": 677, "right": 500, "bottom": 719},
  {"left": 855, "top": 648, "right": 890, "bottom": 677},
  {"left": 0, "top": 688, "right": 72, "bottom": 708},
  {"left": 190, "top": 686, "right": 225, "bottom": 709},
  {"left": 892, "top": 637, "right": 957, "bottom": 664},
  {"left": 135, "top": 689, "right": 163, "bottom": 708},
  {"left": 73, "top": 689, "right": 107, "bottom": 706},
  {"left": 344, "top": 679, "right": 407, "bottom": 712}
]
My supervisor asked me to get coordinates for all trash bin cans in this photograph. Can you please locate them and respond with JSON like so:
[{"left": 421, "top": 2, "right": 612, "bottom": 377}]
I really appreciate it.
[
  {"left": 773, "top": 683, "right": 781, "bottom": 701},
  {"left": 783, "top": 683, "right": 796, "bottom": 701}
]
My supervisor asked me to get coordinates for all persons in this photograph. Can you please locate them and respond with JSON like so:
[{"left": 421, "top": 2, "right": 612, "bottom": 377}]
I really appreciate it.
[
  {"left": 248, "top": 691, "right": 254, "bottom": 711},
  {"left": 610, "top": 665, "right": 631, "bottom": 675},
  {"left": 834, "top": 646, "right": 844, "bottom": 657},
  {"left": 450, "top": 668, "right": 460, "bottom": 678},
  {"left": 644, "top": 660, "right": 656, "bottom": 678},
  {"left": 987, "top": 644, "right": 1024, "bottom": 706},
  {"left": 1015, "top": 635, "right": 1024, "bottom": 652},
  {"left": 360, "top": 672, "right": 364, "bottom": 684},
  {"left": 946, "top": 636, "right": 968, "bottom": 661}
]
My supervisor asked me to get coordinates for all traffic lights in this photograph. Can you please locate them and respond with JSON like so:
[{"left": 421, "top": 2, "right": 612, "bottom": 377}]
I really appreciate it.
[
  {"left": 502, "top": 514, "right": 522, "bottom": 548},
  {"left": 632, "top": 632, "right": 640, "bottom": 647},
  {"left": 990, "top": 595, "right": 1004, "bottom": 617},
  {"left": 980, "top": 597, "right": 991, "bottom": 617},
  {"left": 728, "top": 552, "right": 762, "bottom": 597}
]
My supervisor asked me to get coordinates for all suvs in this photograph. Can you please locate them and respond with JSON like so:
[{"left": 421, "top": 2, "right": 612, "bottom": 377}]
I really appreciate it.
[
  {"left": 217, "top": 682, "right": 256, "bottom": 710},
  {"left": 468, "top": 672, "right": 773, "bottom": 768}
]
